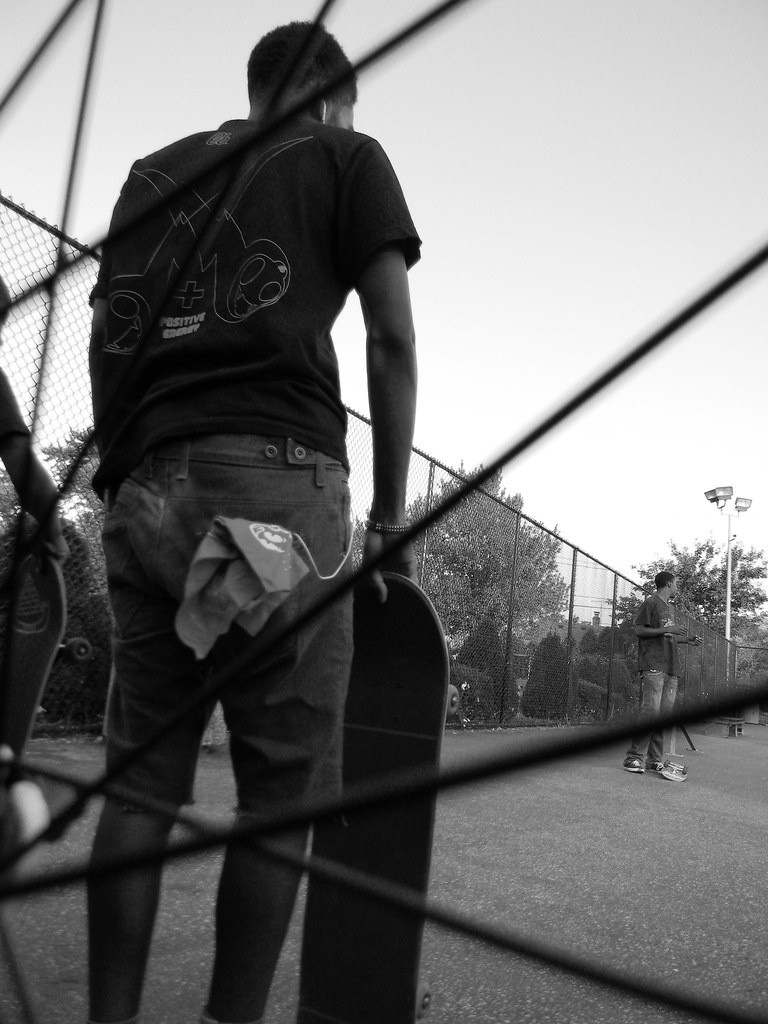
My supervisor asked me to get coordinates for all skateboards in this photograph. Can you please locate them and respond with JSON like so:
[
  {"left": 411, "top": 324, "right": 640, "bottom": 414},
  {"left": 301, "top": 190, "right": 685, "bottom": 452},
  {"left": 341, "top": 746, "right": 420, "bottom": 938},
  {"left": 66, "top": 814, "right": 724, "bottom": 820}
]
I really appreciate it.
[
  {"left": 661, "top": 758, "right": 689, "bottom": 782},
  {"left": 294, "top": 569, "right": 460, "bottom": 1024},
  {"left": 2, "top": 542, "right": 67, "bottom": 760}
]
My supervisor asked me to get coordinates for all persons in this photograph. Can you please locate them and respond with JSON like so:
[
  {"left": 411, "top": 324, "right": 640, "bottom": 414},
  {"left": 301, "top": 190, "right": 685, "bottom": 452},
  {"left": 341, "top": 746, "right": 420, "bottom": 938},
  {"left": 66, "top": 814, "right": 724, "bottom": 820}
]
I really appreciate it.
[
  {"left": 623, "top": 570, "right": 704, "bottom": 774},
  {"left": 0, "top": 276, "right": 70, "bottom": 561},
  {"left": 85, "top": 21, "right": 419, "bottom": 1022}
]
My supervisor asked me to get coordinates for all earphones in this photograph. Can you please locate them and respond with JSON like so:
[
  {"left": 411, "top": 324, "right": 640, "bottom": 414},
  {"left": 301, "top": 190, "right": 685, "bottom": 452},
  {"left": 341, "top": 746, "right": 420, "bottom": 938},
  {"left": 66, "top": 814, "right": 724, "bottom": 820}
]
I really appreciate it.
[{"left": 319, "top": 99, "right": 328, "bottom": 126}]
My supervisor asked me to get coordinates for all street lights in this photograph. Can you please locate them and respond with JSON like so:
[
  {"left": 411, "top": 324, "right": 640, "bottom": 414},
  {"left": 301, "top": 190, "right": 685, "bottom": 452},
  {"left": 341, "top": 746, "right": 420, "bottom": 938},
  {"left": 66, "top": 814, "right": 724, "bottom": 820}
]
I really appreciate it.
[{"left": 706, "top": 483, "right": 755, "bottom": 690}]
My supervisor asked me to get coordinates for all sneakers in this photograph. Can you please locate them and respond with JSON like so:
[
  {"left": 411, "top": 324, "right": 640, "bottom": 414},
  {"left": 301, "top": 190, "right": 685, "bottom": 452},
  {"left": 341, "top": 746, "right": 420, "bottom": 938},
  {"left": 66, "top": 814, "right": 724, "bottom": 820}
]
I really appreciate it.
[
  {"left": 623, "top": 759, "right": 645, "bottom": 773},
  {"left": 645, "top": 761, "right": 666, "bottom": 774}
]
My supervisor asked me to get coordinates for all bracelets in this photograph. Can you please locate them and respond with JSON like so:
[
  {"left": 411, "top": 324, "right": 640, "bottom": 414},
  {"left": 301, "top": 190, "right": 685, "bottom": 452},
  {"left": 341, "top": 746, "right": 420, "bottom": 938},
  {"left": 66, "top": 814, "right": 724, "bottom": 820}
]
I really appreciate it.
[{"left": 362, "top": 520, "right": 412, "bottom": 535}]
